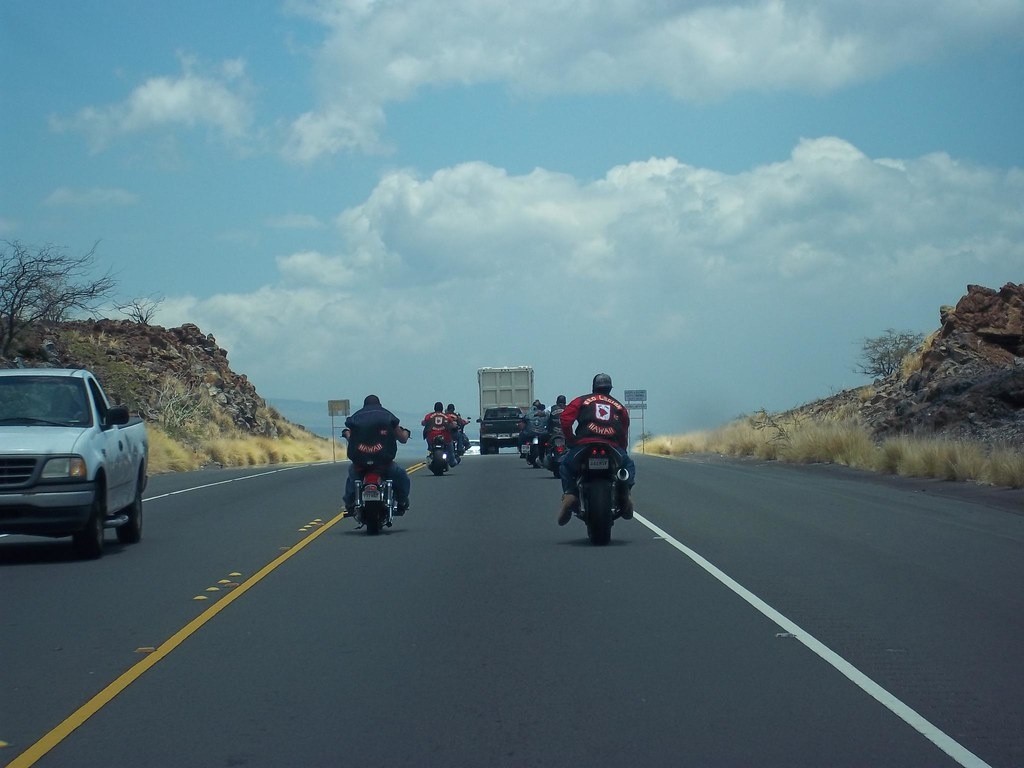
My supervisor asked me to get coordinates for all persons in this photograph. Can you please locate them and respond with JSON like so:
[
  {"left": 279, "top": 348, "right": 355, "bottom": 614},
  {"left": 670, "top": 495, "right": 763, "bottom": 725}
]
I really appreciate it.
[
  {"left": 558, "top": 374, "right": 636, "bottom": 526},
  {"left": 515, "top": 399, "right": 552, "bottom": 460},
  {"left": 533, "top": 394, "right": 571, "bottom": 457},
  {"left": 421, "top": 401, "right": 471, "bottom": 470},
  {"left": 341, "top": 394, "right": 410, "bottom": 515}
]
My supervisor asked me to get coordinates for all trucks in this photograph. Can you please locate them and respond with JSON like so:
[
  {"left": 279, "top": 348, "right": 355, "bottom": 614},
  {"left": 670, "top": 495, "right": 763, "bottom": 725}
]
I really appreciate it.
[{"left": 477, "top": 367, "right": 535, "bottom": 422}]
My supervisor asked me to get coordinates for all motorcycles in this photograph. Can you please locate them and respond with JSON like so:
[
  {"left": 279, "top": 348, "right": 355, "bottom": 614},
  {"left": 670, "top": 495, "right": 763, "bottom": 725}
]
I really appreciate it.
[
  {"left": 516, "top": 418, "right": 570, "bottom": 479},
  {"left": 426, "top": 416, "right": 471, "bottom": 476},
  {"left": 567, "top": 441, "right": 626, "bottom": 545},
  {"left": 340, "top": 426, "right": 413, "bottom": 536}
]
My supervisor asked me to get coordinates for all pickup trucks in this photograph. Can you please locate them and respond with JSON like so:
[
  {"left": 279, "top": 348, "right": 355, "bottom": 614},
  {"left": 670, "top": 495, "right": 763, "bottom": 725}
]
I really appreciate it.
[
  {"left": 476, "top": 405, "right": 526, "bottom": 456},
  {"left": 1, "top": 367, "right": 148, "bottom": 559}
]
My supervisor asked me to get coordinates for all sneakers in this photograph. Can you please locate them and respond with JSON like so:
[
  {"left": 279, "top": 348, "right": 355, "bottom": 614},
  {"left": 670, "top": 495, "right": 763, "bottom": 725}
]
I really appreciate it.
[
  {"left": 558, "top": 493, "right": 576, "bottom": 526},
  {"left": 621, "top": 495, "right": 633, "bottom": 520}
]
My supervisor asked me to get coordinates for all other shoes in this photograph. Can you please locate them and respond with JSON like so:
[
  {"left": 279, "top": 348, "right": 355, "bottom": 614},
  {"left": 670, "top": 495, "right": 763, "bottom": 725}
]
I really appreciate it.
[
  {"left": 451, "top": 458, "right": 461, "bottom": 468},
  {"left": 397, "top": 498, "right": 410, "bottom": 513},
  {"left": 520, "top": 454, "right": 525, "bottom": 458},
  {"left": 346, "top": 506, "right": 355, "bottom": 515}
]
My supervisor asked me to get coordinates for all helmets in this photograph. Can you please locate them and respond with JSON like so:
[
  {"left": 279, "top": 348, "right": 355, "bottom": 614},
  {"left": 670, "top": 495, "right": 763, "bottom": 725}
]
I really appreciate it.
[
  {"left": 593, "top": 373, "right": 612, "bottom": 393},
  {"left": 447, "top": 404, "right": 455, "bottom": 411},
  {"left": 557, "top": 395, "right": 566, "bottom": 404},
  {"left": 434, "top": 402, "right": 443, "bottom": 411}
]
